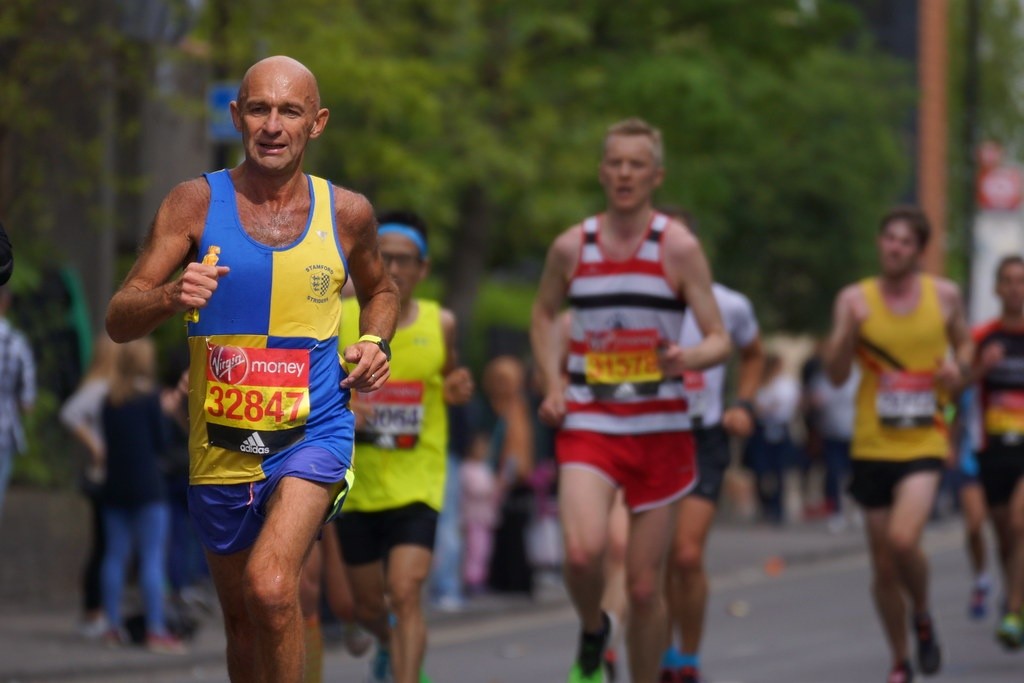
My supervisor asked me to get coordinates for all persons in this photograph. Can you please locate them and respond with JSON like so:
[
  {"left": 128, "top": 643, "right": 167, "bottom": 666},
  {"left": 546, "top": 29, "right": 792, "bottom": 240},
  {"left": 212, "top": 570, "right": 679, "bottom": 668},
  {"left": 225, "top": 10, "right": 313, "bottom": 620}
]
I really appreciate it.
[
  {"left": 0, "top": 226, "right": 211, "bottom": 683},
  {"left": 300, "top": 214, "right": 470, "bottom": 683},
  {"left": 762, "top": 209, "right": 1024, "bottom": 683},
  {"left": 471, "top": 117, "right": 764, "bottom": 683},
  {"left": 106, "top": 54, "right": 400, "bottom": 683}
]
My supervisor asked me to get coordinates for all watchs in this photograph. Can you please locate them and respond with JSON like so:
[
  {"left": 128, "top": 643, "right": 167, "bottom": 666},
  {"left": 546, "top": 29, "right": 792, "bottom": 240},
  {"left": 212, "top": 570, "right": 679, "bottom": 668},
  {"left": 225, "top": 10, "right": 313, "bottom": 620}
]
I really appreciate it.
[{"left": 358, "top": 334, "right": 391, "bottom": 361}]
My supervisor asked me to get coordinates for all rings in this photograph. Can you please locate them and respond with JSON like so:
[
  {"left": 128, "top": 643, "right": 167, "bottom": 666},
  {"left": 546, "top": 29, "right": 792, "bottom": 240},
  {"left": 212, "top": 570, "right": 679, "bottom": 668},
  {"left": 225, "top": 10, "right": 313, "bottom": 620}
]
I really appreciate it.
[{"left": 371, "top": 374, "right": 377, "bottom": 381}]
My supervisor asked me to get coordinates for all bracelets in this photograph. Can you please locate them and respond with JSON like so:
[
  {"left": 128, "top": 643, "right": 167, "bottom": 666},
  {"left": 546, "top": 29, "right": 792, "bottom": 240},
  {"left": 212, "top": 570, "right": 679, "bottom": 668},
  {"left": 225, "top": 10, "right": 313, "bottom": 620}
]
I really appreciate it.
[{"left": 737, "top": 399, "right": 754, "bottom": 413}]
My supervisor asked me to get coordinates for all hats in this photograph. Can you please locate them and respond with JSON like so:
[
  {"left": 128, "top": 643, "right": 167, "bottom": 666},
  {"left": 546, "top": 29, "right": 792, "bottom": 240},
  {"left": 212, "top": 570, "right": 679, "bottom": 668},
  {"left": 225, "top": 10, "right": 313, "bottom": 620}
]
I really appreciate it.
[{"left": 0, "top": 224, "right": 13, "bottom": 287}]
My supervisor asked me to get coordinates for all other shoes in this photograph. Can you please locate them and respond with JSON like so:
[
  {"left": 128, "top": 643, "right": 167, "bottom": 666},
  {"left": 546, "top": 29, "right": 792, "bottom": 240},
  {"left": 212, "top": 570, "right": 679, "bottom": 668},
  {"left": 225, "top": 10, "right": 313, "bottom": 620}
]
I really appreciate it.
[
  {"left": 995, "top": 600, "right": 1024, "bottom": 648},
  {"left": 78, "top": 611, "right": 187, "bottom": 655},
  {"left": 968, "top": 577, "right": 989, "bottom": 618},
  {"left": 912, "top": 612, "right": 940, "bottom": 676},
  {"left": 887, "top": 659, "right": 914, "bottom": 683},
  {"left": 570, "top": 608, "right": 611, "bottom": 683},
  {"left": 663, "top": 648, "right": 701, "bottom": 683}
]
